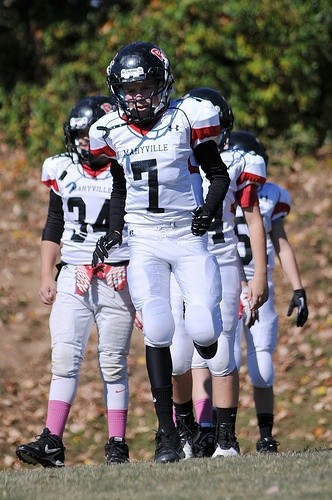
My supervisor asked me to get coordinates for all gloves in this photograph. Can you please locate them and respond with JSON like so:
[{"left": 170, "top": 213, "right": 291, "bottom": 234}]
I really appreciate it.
[
  {"left": 238, "top": 287, "right": 259, "bottom": 328},
  {"left": 287, "top": 288, "right": 308, "bottom": 328},
  {"left": 74, "top": 264, "right": 97, "bottom": 296},
  {"left": 96, "top": 262, "right": 127, "bottom": 290},
  {"left": 132, "top": 312, "right": 143, "bottom": 330},
  {"left": 190, "top": 205, "right": 212, "bottom": 237},
  {"left": 91, "top": 233, "right": 123, "bottom": 268}
]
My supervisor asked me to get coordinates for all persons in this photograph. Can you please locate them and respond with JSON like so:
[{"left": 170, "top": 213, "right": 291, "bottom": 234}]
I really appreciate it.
[
  {"left": 89, "top": 43, "right": 231, "bottom": 463},
  {"left": 15, "top": 96, "right": 136, "bottom": 469},
  {"left": 173, "top": 131, "right": 308, "bottom": 458},
  {"left": 170, "top": 90, "right": 269, "bottom": 460}
]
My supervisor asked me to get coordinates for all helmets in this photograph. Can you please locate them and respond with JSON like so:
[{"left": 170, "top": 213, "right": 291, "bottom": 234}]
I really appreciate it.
[
  {"left": 228, "top": 130, "right": 268, "bottom": 167},
  {"left": 187, "top": 88, "right": 233, "bottom": 152},
  {"left": 64, "top": 96, "right": 117, "bottom": 162},
  {"left": 106, "top": 43, "right": 174, "bottom": 126}
]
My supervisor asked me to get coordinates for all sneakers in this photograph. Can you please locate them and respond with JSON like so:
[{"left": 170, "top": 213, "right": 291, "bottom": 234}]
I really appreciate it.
[
  {"left": 174, "top": 412, "right": 195, "bottom": 460},
  {"left": 213, "top": 425, "right": 240, "bottom": 458},
  {"left": 194, "top": 430, "right": 215, "bottom": 458},
  {"left": 15, "top": 428, "right": 65, "bottom": 466},
  {"left": 104, "top": 438, "right": 130, "bottom": 464},
  {"left": 153, "top": 425, "right": 180, "bottom": 463},
  {"left": 257, "top": 437, "right": 278, "bottom": 453}
]
